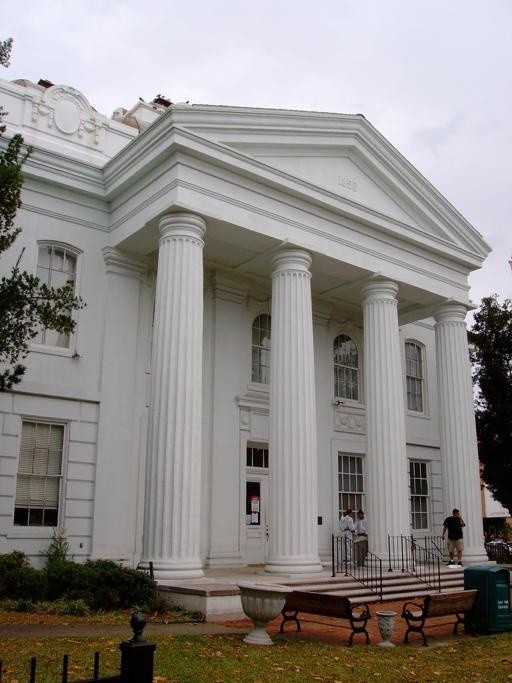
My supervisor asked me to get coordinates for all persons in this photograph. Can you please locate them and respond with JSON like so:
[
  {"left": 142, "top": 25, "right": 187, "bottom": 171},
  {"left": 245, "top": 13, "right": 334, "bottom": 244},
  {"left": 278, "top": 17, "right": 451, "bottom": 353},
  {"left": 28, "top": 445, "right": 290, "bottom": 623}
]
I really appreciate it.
[
  {"left": 441, "top": 508, "right": 466, "bottom": 566},
  {"left": 337, "top": 508, "right": 356, "bottom": 568},
  {"left": 351, "top": 510, "right": 368, "bottom": 568}
]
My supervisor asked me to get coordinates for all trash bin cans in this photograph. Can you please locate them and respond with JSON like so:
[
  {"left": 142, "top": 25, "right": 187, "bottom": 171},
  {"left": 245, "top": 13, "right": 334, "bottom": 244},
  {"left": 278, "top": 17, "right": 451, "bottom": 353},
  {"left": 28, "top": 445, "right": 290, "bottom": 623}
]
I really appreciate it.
[{"left": 464, "top": 565, "right": 512, "bottom": 635}]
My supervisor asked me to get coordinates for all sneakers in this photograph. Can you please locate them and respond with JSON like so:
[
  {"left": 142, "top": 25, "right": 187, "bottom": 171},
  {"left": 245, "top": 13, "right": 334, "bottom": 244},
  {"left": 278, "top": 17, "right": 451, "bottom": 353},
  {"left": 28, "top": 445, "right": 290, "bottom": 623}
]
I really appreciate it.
[{"left": 447, "top": 560, "right": 463, "bottom": 566}]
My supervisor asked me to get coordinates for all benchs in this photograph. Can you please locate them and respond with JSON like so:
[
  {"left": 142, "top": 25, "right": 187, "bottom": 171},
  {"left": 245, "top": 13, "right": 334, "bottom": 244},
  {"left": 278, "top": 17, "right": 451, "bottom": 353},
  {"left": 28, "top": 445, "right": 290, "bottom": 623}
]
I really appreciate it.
[
  {"left": 278, "top": 587, "right": 372, "bottom": 647},
  {"left": 400, "top": 589, "right": 480, "bottom": 648}
]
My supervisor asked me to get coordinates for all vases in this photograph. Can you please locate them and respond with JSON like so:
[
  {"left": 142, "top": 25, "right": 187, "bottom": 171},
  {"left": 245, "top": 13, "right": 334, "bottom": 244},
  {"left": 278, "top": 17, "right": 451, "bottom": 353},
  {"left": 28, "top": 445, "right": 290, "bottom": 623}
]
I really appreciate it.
[
  {"left": 235, "top": 581, "right": 295, "bottom": 647},
  {"left": 375, "top": 611, "right": 398, "bottom": 648}
]
